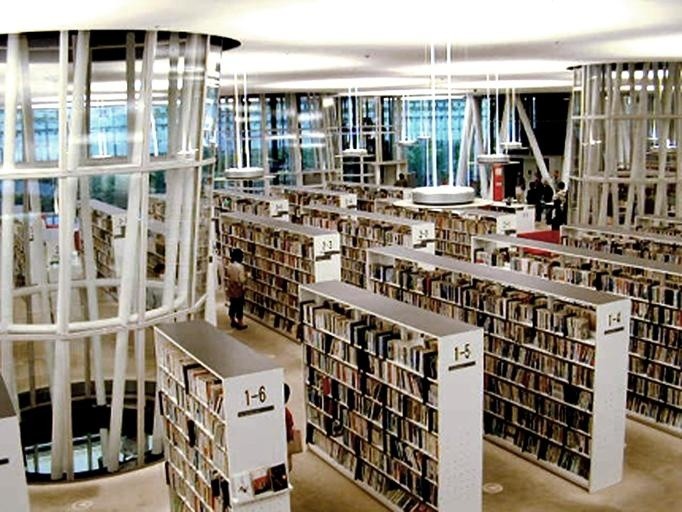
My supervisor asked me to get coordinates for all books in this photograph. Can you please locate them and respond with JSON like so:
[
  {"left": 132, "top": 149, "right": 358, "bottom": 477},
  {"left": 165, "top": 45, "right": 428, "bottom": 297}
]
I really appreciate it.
[{"left": 77, "top": 179, "right": 681, "bottom": 509}]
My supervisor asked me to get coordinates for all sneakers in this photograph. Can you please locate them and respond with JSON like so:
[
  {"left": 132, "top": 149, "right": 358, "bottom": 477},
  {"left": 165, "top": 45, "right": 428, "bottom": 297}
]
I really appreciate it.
[{"left": 230, "top": 321, "right": 248, "bottom": 331}]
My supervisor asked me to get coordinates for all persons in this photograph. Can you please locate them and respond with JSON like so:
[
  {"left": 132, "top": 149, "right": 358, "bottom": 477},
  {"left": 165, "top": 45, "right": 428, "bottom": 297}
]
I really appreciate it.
[{"left": 393, "top": 173, "right": 409, "bottom": 187}]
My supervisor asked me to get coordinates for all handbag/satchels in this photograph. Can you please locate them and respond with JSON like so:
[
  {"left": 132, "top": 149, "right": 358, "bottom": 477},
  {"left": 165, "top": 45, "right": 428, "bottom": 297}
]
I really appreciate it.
[{"left": 287, "top": 429, "right": 304, "bottom": 456}]
